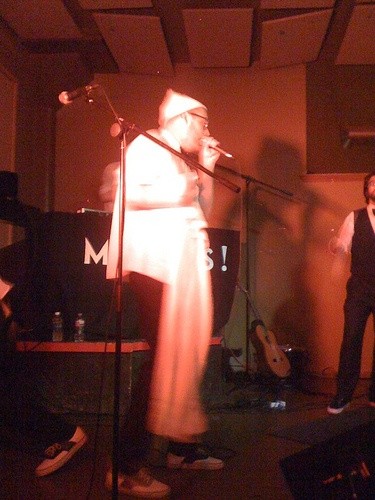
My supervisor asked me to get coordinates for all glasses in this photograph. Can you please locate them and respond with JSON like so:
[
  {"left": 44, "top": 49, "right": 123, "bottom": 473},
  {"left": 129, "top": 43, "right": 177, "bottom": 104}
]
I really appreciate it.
[{"left": 190, "top": 112, "right": 210, "bottom": 128}]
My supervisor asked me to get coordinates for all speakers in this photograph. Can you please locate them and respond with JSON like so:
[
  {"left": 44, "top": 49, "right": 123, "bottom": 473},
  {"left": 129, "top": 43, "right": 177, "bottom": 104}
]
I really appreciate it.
[{"left": 278, "top": 418, "right": 375, "bottom": 500}]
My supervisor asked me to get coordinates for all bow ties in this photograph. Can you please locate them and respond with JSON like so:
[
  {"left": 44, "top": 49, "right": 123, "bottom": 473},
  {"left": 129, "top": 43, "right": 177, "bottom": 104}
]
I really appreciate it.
[{"left": 373, "top": 209, "right": 375, "bottom": 215}]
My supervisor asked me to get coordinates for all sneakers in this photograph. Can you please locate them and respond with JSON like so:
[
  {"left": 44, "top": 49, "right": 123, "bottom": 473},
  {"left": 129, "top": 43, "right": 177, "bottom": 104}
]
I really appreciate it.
[
  {"left": 166, "top": 447, "right": 224, "bottom": 470},
  {"left": 104, "top": 462, "right": 172, "bottom": 499},
  {"left": 34, "top": 424, "right": 89, "bottom": 477}
]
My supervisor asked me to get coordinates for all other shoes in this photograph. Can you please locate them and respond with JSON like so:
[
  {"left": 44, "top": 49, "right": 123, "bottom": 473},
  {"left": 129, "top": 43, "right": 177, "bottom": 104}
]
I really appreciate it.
[
  {"left": 326, "top": 394, "right": 351, "bottom": 415},
  {"left": 367, "top": 392, "right": 375, "bottom": 408}
]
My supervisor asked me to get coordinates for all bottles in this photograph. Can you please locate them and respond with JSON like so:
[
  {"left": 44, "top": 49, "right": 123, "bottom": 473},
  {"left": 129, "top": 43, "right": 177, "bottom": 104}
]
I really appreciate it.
[
  {"left": 52, "top": 312, "right": 63, "bottom": 342},
  {"left": 74, "top": 312, "right": 86, "bottom": 342}
]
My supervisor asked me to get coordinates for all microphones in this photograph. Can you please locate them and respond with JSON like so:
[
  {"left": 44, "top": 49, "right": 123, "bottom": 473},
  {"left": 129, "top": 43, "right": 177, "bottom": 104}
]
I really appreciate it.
[
  {"left": 210, "top": 145, "right": 234, "bottom": 160},
  {"left": 59, "top": 83, "right": 99, "bottom": 104}
]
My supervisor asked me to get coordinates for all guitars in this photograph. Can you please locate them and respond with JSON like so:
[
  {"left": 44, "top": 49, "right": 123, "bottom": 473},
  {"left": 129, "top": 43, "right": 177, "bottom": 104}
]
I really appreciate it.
[{"left": 236, "top": 281, "right": 293, "bottom": 379}]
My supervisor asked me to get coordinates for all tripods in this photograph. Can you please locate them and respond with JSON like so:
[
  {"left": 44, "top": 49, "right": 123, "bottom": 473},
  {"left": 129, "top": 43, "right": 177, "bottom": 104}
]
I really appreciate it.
[{"left": 215, "top": 163, "right": 294, "bottom": 395}]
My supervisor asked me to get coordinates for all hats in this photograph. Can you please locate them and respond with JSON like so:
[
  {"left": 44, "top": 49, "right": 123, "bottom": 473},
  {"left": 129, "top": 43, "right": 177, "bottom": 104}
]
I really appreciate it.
[{"left": 158, "top": 88, "right": 208, "bottom": 125}]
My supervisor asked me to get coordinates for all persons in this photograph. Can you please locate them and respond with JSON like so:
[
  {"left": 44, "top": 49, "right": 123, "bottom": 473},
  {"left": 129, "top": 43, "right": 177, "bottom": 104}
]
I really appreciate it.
[
  {"left": 0, "top": 279, "right": 88, "bottom": 477},
  {"left": 104, "top": 93, "right": 225, "bottom": 496},
  {"left": 327, "top": 172, "right": 375, "bottom": 414}
]
